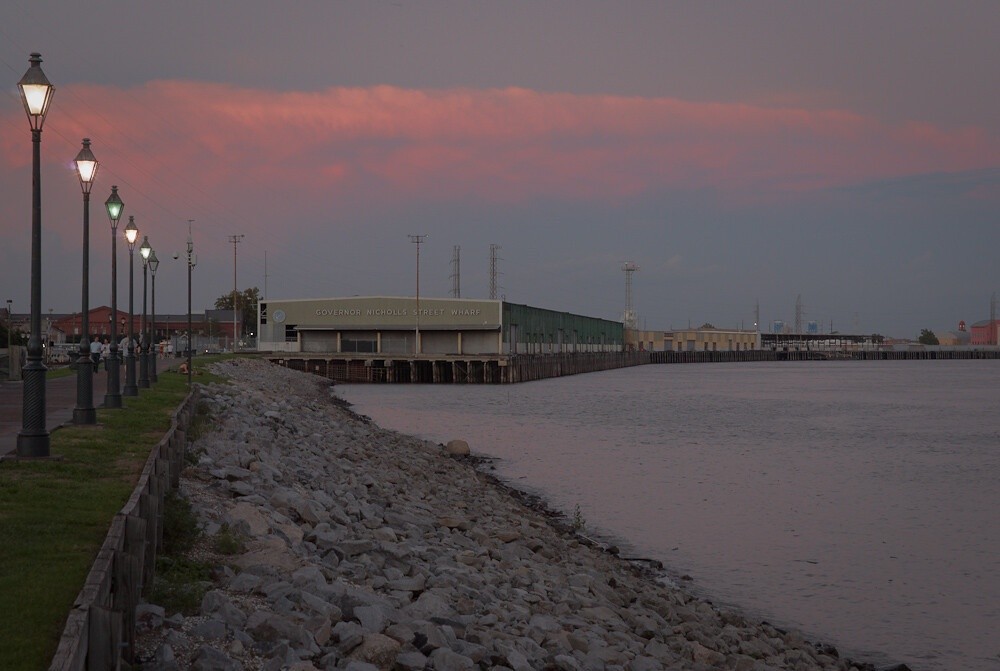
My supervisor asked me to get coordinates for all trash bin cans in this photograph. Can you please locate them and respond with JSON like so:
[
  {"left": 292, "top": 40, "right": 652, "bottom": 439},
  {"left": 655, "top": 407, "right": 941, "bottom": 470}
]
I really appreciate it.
[{"left": 8, "top": 345, "right": 28, "bottom": 381}]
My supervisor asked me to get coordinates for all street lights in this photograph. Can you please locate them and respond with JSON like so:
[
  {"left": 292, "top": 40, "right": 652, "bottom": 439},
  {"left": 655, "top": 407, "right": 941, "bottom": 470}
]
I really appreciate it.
[
  {"left": 137, "top": 235, "right": 151, "bottom": 388},
  {"left": 5, "top": 298, "right": 13, "bottom": 346},
  {"left": 148, "top": 250, "right": 159, "bottom": 383},
  {"left": 123, "top": 215, "right": 139, "bottom": 399},
  {"left": 71, "top": 136, "right": 108, "bottom": 427},
  {"left": 104, "top": 183, "right": 127, "bottom": 408},
  {"left": 121, "top": 317, "right": 125, "bottom": 339},
  {"left": 15, "top": 51, "right": 55, "bottom": 457}
]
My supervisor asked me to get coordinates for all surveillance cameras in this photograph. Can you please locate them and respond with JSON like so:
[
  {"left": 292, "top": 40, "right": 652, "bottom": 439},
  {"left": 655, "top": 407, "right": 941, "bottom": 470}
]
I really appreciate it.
[{"left": 173, "top": 252, "right": 179, "bottom": 259}]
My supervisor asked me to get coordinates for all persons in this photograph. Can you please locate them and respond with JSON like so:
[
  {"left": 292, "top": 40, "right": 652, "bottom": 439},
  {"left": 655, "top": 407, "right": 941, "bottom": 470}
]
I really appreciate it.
[
  {"left": 90, "top": 336, "right": 103, "bottom": 374},
  {"left": 159, "top": 344, "right": 173, "bottom": 359},
  {"left": 101, "top": 339, "right": 110, "bottom": 371},
  {"left": 120, "top": 330, "right": 137, "bottom": 379},
  {"left": 180, "top": 359, "right": 199, "bottom": 374}
]
